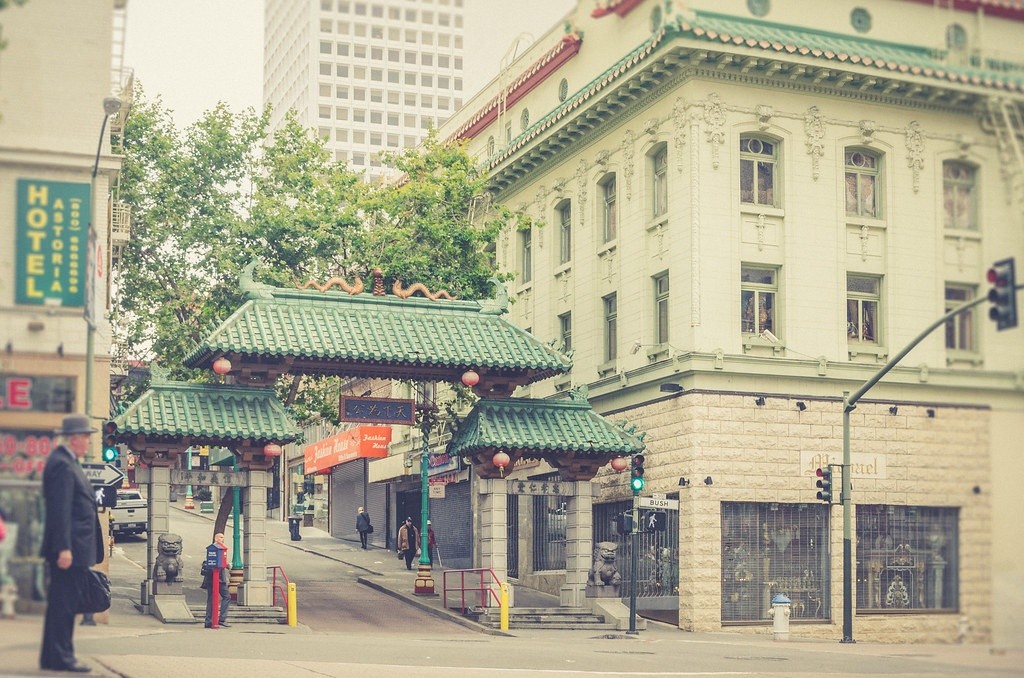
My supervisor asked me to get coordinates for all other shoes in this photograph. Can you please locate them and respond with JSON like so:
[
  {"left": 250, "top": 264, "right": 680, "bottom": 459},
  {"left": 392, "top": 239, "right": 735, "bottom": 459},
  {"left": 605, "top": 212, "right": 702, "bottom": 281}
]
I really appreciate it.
[
  {"left": 64, "top": 663, "right": 91, "bottom": 673},
  {"left": 218, "top": 621, "right": 231, "bottom": 627}
]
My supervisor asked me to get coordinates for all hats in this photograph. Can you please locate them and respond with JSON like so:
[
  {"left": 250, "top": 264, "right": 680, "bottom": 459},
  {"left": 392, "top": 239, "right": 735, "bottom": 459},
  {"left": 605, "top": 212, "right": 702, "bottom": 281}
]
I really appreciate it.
[
  {"left": 358, "top": 507, "right": 364, "bottom": 513},
  {"left": 54, "top": 417, "right": 99, "bottom": 434},
  {"left": 427, "top": 520, "right": 431, "bottom": 524}
]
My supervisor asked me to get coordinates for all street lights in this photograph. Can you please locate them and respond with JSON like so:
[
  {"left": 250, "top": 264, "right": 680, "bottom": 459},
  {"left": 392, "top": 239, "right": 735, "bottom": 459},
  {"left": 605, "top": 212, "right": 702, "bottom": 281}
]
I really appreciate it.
[{"left": 81, "top": 93, "right": 122, "bottom": 626}]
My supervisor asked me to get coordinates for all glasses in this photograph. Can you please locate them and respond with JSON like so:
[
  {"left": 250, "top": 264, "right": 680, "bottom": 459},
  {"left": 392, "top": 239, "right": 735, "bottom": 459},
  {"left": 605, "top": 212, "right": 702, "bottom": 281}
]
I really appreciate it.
[{"left": 408, "top": 520, "right": 412, "bottom": 522}]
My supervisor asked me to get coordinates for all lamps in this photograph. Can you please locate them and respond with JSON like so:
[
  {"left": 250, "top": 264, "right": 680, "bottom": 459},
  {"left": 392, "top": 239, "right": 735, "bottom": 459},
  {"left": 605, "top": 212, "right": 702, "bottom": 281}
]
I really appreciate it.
[
  {"left": 679, "top": 477, "right": 689, "bottom": 487},
  {"left": 796, "top": 401, "right": 806, "bottom": 411},
  {"left": 660, "top": 384, "right": 683, "bottom": 392},
  {"left": 756, "top": 397, "right": 765, "bottom": 405},
  {"left": 927, "top": 409, "right": 934, "bottom": 417},
  {"left": 703, "top": 477, "right": 713, "bottom": 485},
  {"left": 890, "top": 407, "right": 897, "bottom": 414}
]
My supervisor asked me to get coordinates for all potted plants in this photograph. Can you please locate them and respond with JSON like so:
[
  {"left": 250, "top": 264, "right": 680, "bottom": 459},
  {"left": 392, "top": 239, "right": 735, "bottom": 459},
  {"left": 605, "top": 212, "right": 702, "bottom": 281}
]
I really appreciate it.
[{"left": 197, "top": 488, "right": 214, "bottom": 514}]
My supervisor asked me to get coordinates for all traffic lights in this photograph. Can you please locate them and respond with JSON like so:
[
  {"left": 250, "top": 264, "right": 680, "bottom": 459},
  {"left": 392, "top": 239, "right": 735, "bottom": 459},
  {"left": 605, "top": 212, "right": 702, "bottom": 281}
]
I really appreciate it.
[
  {"left": 91, "top": 483, "right": 115, "bottom": 507},
  {"left": 102, "top": 420, "right": 118, "bottom": 461},
  {"left": 631, "top": 454, "right": 644, "bottom": 491},
  {"left": 987, "top": 258, "right": 1018, "bottom": 330},
  {"left": 646, "top": 512, "right": 667, "bottom": 532},
  {"left": 816, "top": 467, "right": 832, "bottom": 501}
]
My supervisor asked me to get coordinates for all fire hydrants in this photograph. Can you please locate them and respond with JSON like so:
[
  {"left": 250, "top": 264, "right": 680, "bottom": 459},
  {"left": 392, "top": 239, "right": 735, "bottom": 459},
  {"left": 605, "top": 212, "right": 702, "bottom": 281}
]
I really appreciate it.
[{"left": 769, "top": 594, "right": 792, "bottom": 641}]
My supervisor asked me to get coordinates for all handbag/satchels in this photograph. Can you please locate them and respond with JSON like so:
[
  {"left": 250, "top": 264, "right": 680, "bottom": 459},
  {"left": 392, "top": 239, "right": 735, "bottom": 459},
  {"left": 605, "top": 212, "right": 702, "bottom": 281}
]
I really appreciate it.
[
  {"left": 367, "top": 525, "right": 373, "bottom": 533},
  {"left": 75, "top": 570, "right": 111, "bottom": 613},
  {"left": 397, "top": 550, "right": 404, "bottom": 560},
  {"left": 415, "top": 548, "right": 421, "bottom": 557}
]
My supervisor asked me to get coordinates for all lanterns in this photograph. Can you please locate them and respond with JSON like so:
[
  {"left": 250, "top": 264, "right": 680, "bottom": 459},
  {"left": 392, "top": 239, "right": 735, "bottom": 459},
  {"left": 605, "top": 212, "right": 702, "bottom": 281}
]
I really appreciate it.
[
  {"left": 611, "top": 455, "right": 627, "bottom": 471},
  {"left": 213, "top": 357, "right": 231, "bottom": 376},
  {"left": 263, "top": 442, "right": 281, "bottom": 458},
  {"left": 492, "top": 450, "right": 510, "bottom": 471},
  {"left": 462, "top": 370, "right": 479, "bottom": 388}
]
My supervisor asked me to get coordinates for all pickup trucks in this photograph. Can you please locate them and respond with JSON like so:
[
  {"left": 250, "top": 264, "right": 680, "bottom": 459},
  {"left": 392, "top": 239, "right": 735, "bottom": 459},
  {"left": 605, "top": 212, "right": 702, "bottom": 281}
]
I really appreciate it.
[{"left": 110, "top": 490, "right": 147, "bottom": 539}]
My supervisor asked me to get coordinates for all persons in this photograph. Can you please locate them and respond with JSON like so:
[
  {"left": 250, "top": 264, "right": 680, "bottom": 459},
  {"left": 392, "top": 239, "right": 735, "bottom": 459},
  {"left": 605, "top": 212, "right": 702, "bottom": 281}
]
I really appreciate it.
[
  {"left": 39, "top": 416, "right": 104, "bottom": 673},
  {"left": 398, "top": 517, "right": 419, "bottom": 570},
  {"left": 418, "top": 520, "right": 436, "bottom": 570},
  {"left": 200, "top": 533, "right": 231, "bottom": 628},
  {"left": 355, "top": 507, "right": 370, "bottom": 549}
]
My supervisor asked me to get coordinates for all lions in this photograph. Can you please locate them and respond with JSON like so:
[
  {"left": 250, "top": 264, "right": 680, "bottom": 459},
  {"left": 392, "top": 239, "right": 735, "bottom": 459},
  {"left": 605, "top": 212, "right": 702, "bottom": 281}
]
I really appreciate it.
[
  {"left": 154, "top": 533, "right": 184, "bottom": 581},
  {"left": 586, "top": 541, "right": 622, "bottom": 586}
]
